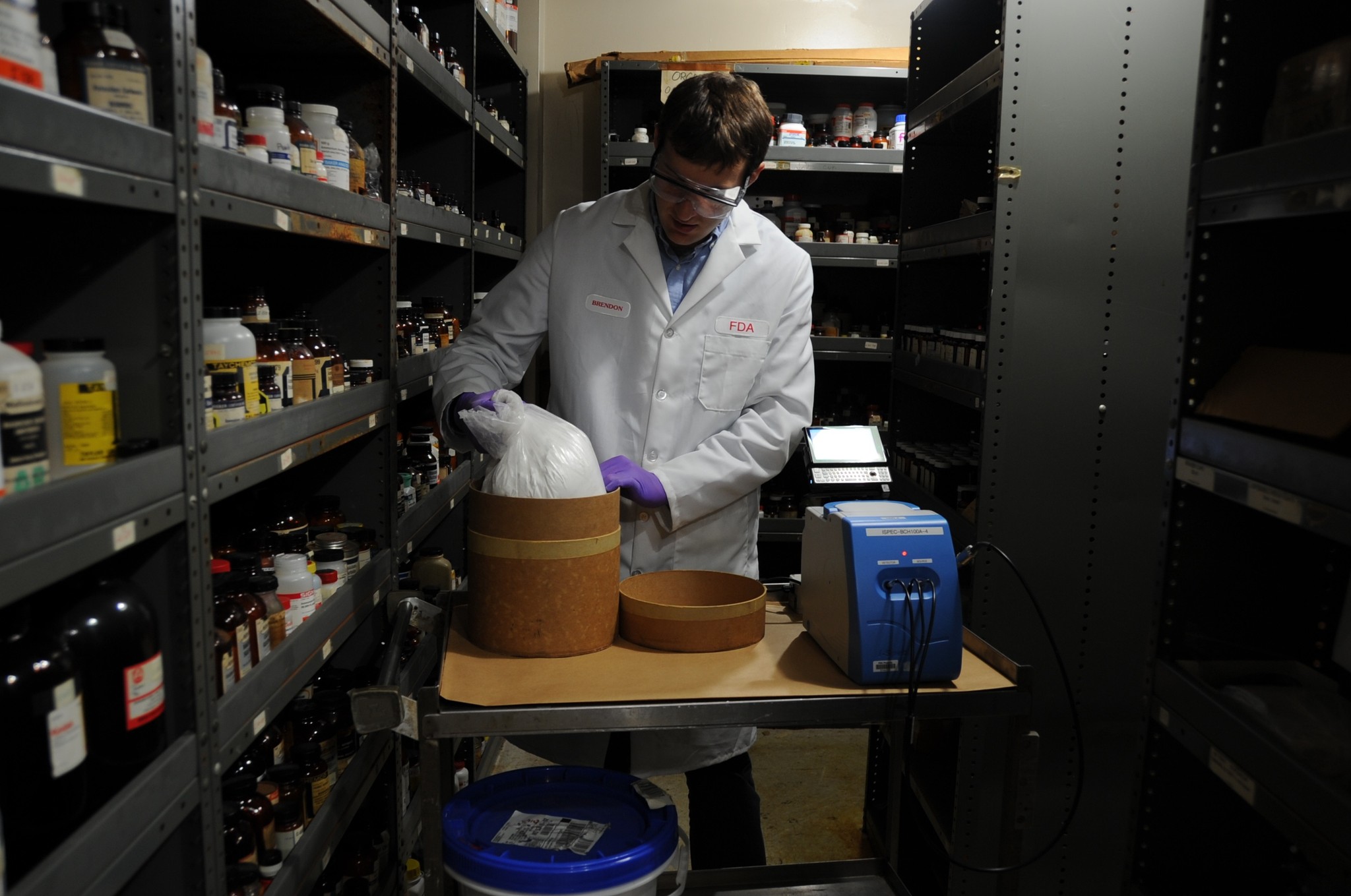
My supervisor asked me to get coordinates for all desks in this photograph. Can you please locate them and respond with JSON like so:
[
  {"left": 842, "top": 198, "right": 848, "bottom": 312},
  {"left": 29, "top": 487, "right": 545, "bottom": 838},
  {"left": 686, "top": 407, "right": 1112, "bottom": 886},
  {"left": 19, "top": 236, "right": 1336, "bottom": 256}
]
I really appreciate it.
[{"left": 410, "top": 592, "right": 1024, "bottom": 896}]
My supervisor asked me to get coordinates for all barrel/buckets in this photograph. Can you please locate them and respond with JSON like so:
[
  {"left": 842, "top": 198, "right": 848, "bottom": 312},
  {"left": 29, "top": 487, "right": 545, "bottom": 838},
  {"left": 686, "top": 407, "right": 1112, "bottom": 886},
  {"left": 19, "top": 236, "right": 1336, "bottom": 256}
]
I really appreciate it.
[{"left": 441, "top": 765, "right": 691, "bottom": 896}]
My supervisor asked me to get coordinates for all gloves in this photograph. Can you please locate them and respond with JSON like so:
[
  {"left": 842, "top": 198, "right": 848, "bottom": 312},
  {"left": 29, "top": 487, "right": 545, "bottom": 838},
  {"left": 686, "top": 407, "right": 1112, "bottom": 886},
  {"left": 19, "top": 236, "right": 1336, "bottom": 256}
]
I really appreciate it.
[
  {"left": 448, "top": 390, "right": 527, "bottom": 454},
  {"left": 599, "top": 455, "right": 668, "bottom": 509}
]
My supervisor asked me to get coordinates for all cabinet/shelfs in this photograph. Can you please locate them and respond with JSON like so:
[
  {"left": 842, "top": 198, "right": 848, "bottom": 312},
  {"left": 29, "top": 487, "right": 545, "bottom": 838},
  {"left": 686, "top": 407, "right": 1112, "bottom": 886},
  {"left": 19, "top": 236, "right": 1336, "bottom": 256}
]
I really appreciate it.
[
  {"left": 893, "top": 0, "right": 1205, "bottom": 896},
  {"left": 598, "top": 58, "right": 909, "bottom": 581},
  {"left": 1145, "top": 0, "right": 1351, "bottom": 893},
  {"left": 0, "top": 0, "right": 532, "bottom": 896}
]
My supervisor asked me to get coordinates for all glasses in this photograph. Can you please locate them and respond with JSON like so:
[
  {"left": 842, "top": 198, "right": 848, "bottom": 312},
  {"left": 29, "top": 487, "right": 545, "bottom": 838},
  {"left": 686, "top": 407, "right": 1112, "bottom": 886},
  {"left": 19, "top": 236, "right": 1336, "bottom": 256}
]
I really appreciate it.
[{"left": 649, "top": 146, "right": 750, "bottom": 218}]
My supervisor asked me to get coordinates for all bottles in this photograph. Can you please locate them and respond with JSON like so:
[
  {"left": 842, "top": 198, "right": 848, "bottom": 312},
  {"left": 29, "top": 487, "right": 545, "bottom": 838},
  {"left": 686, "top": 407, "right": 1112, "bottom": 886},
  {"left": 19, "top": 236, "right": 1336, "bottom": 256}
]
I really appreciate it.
[
  {"left": 631, "top": 102, "right": 906, "bottom": 148},
  {"left": 1, "top": 0, "right": 153, "bottom": 127},
  {"left": 396, "top": 169, "right": 505, "bottom": 231},
  {"left": 0, "top": 561, "right": 167, "bottom": 894},
  {"left": 475, "top": 736, "right": 489, "bottom": 765},
  {"left": 395, "top": 413, "right": 457, "bottom": 518},
  {"left": 405, "top": 859, "right": 425, "bottom": 896},
  {"left": 407, "top": 6, "right": 520, "bottom": 141},
  {"left": 222, "top": 294, "right": 350, "bottom": 419},
  {"left": 759, "top": 194, "right": 898, "bottom": 245},
  {"left": 223, "top": 600, "right": 389, "bottom": 896},
  {"left": 350, "top": 359, "right": 374, "bottom": 387},
  {"left": 313, "top": 782, "right": 398, "bottom": 896},
  {"left": 396, "top": 295, "right": 463, "bottom": 358},
  {"left": 402, "top": 737, "right": 421, "bottom": 816},
  {"left": 474, "top": 292, "right": 489, "bottom": 308},
  {"left": 202, "top": 306, "right": 260, "bottom": 433},
  {"left": 454, "top": 760, "right": 468, "bottom": 794},
  {"left": 398, "top": 546, "right": 462, "bottom": 606},
  {"left": 759, "top": 307, "right": 987, "bottom": 525},
  {"left": 0, "top": 319, "right": 122, "bottom": 502},
  {"left": 209, "top": 493, "right": 379, "bottom": 700},
  {"left": 400, "top": 625, "right": 425, "bottom": 670},
  {"left": 976, "top": 196, "right": 991, "bottom": 214},
  {"left": 195, "top": 46, "right": 382, "bottom": 201}
]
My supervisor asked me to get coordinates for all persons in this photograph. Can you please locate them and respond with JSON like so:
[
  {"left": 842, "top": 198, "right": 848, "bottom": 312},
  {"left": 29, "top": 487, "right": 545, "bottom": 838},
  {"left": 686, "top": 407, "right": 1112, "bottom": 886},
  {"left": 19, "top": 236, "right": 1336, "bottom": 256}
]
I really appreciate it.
[{"left": 434, "top": 73, "right": 814, "bottom": 869}]
